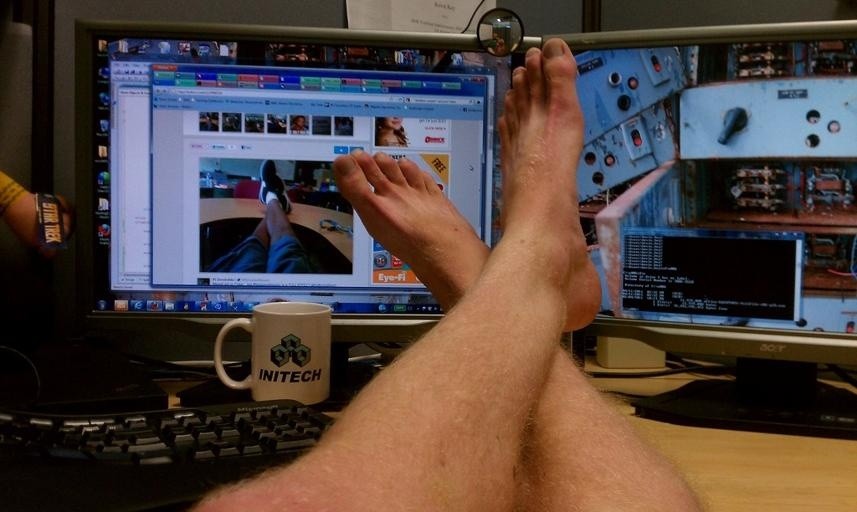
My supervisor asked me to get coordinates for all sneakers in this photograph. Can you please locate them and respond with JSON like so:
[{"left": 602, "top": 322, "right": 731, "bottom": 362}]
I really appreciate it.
[{"left": 259, "top": 160, "right": 293, "bottom": 214}]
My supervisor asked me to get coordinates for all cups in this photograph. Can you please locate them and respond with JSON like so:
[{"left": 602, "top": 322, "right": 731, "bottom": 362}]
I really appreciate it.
[{"left": 214, "top": 300, "right": 334, "bottom": 407}]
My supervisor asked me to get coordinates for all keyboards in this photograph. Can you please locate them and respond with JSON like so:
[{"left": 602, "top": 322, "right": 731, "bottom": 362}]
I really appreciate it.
[{"left": 0, "top": 398, "right": 337, "bottom": 504}]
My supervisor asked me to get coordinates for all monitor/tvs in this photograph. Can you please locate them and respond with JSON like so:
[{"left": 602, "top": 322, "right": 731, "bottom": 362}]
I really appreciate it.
[
  {"left": 617, "top": 226, "right": 805, "bottom": 326},
  {"left": 71, "top": 16, "right": 545, "bottom": 412},
  {"left": 540, "top": 19, "right": 857, "bottom": 441}
]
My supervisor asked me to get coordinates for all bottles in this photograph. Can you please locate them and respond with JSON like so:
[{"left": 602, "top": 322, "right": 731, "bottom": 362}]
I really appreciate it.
[{"left": 206, "top": 171, "right": 213, "bottom": 188}]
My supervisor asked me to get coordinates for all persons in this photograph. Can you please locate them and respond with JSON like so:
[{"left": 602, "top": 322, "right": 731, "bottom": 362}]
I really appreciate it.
[
  {"left": 200, "top": 159, "right": 313, "bottom": 274},
  {"left": 0, "top": 168, "right": 76, "bottom": 262},
  {"left": 289, "top": 116, "right": 307, "bottom": 132},
  {"left": 374, "top": 116, "right": 407, "bottom": 146},
  {"left": 183, "top": 35, "right": 702, "bottom": 510}
]
